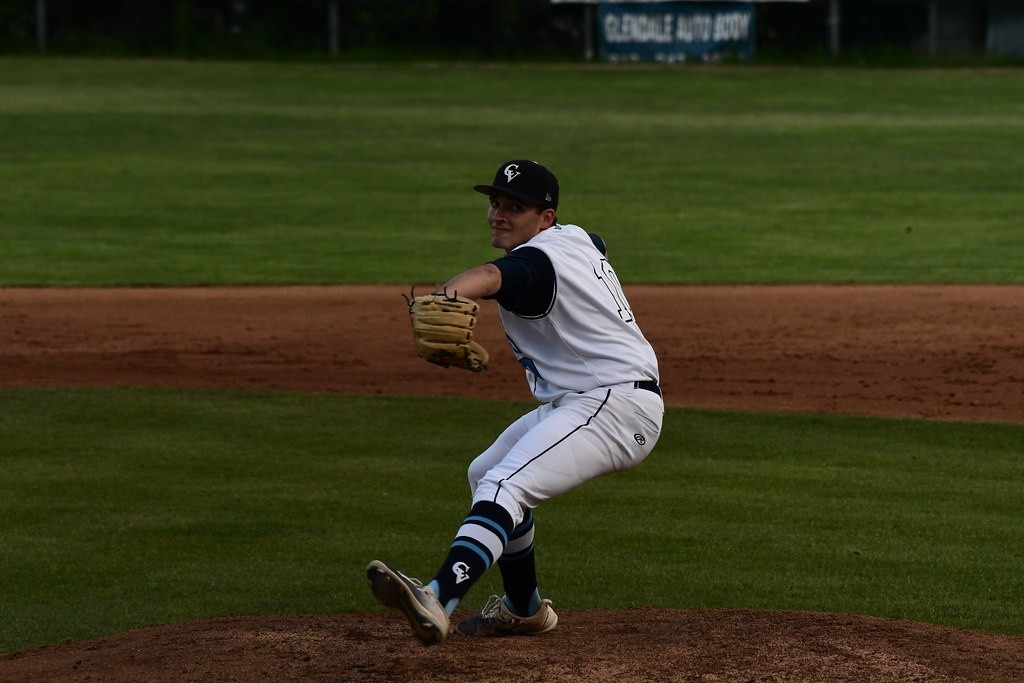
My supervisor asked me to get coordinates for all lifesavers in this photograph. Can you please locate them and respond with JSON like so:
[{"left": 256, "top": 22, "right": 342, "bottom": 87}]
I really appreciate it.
[{"left": 401, "top": 284, "right": 490, "bottom": 374}]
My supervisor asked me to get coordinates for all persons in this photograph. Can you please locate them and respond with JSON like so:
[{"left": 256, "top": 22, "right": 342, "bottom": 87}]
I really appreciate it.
[{"left": 366, "top": 158, "right": 668, "bottom": 648}]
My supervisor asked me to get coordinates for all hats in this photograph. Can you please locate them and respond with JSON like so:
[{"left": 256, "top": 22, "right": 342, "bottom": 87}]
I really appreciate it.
[{"left": 472, "top": 160, "right": 559, "bottom": 212}]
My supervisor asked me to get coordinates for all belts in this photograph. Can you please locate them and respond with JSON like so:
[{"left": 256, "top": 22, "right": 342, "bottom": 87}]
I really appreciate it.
[{"left": 635, "top": 381, "right": 662, "bottom": 398}]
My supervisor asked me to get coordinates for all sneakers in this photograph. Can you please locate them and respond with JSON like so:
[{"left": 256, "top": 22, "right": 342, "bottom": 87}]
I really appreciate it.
[
  {"left": 365, "top": 560, "right": 450, "bottom": 646},
  {"left": 455, "top": 594, "right": 559, "bottom": 636}
]
